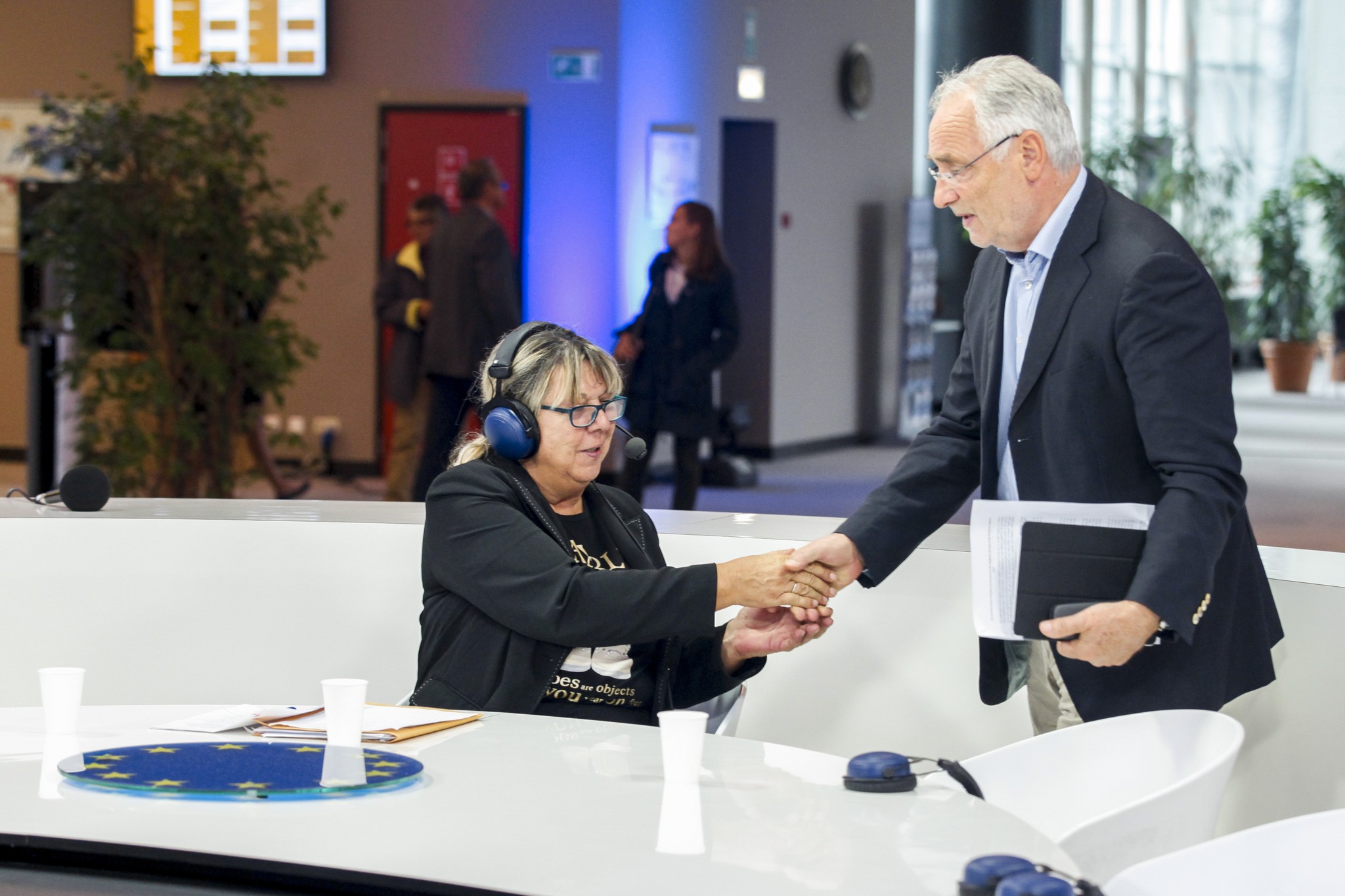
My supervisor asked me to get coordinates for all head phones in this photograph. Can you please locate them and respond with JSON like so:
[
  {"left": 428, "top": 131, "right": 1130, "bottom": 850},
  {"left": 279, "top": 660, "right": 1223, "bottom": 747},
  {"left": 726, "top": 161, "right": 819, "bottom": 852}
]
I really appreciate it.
[{"left": 481, "top": 320, "right": 564, "bottom": 459}]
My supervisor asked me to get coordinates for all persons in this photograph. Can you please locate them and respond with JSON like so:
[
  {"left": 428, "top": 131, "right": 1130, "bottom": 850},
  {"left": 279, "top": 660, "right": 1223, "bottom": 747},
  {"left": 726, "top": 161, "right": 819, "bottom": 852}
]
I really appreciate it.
[
  {"left": 400, "top": 321, "right": 834, "bottom": 727},
  {"left": 412, "top": 157, "right": 520, "bottom": 502},
  {"left": 613, "top": 200, "right": 740, "bottom": 511},
  {"left": 374, "top": 192, "right": 449, "bottom": 502},
  {"left": 767, "top": 55, "right": 1288, "bottom": 736}
]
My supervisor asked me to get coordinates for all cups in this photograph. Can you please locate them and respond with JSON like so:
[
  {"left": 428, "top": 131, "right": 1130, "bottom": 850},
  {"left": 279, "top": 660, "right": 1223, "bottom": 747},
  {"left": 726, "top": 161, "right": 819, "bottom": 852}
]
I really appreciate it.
[
  {"left": 36, "top": 665, "right": 88, "bottom": 720},
  {"left": 657, "top": 710, "right": 712, "bottom": 783},
  {"left": 654, "top": 781, "right": 705, "bottom": 856},
  {"left": 319, "top": 742, "right": 367, "bottom": 788},
  {"left": 320, "top": 678, "right": 368, "bottom": 745}
]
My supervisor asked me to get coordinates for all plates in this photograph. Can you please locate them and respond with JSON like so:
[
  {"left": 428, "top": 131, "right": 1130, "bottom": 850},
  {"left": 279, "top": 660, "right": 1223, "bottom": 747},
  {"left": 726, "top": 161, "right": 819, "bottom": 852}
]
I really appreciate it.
[{"left": 57, "top": 742, "right": 424, "bottom": 803}]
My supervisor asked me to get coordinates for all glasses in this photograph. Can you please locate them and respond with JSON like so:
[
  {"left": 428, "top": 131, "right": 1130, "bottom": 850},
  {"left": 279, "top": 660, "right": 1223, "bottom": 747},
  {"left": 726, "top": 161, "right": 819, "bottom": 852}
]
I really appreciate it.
[
  {"left": 927, "top": 133, "right": 1021, "bottom": 187},
  {"left": 541, "top": 394, "right": 627, "bottom": 427}
]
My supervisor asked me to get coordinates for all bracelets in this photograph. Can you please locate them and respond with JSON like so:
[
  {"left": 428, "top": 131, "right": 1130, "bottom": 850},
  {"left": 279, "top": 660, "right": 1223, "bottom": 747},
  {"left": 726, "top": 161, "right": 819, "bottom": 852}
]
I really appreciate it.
[{"left": 1145, "top": 616, "right": 1168, "bottom": 648}]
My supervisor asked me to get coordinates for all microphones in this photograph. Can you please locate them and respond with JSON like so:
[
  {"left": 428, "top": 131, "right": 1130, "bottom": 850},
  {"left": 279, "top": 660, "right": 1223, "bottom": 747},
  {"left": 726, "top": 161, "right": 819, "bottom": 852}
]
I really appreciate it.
[
  {"left": 615, "top": 425, "right": 647, "bottom": 461},
  {"left": 36, "top": 466, "right": 109, "bottom": 512}
]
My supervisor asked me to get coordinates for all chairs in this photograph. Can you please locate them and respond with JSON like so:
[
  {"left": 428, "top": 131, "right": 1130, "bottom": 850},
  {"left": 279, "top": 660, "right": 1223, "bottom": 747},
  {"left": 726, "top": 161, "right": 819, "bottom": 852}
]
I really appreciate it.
[
  {"left": 1101, "top": 807, "right": 1345, "bottom": 895},
  {"left": 921, "top": 705, "right": 1244, "bottom": 882}
]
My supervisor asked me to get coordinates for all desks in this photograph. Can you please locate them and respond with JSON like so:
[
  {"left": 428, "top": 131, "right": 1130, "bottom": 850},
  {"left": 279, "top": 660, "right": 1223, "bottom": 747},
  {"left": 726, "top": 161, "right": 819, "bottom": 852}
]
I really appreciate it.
[{"left": 0, "top": 659, "right": 1097, "bottom": 896}]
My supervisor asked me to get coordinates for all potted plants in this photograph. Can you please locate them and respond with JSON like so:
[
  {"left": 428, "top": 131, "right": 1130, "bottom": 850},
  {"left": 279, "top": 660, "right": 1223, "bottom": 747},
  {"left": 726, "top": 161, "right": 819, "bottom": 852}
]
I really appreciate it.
[{"left": 1250, "top": 193, "right": 1321, "bottom": 392}]
